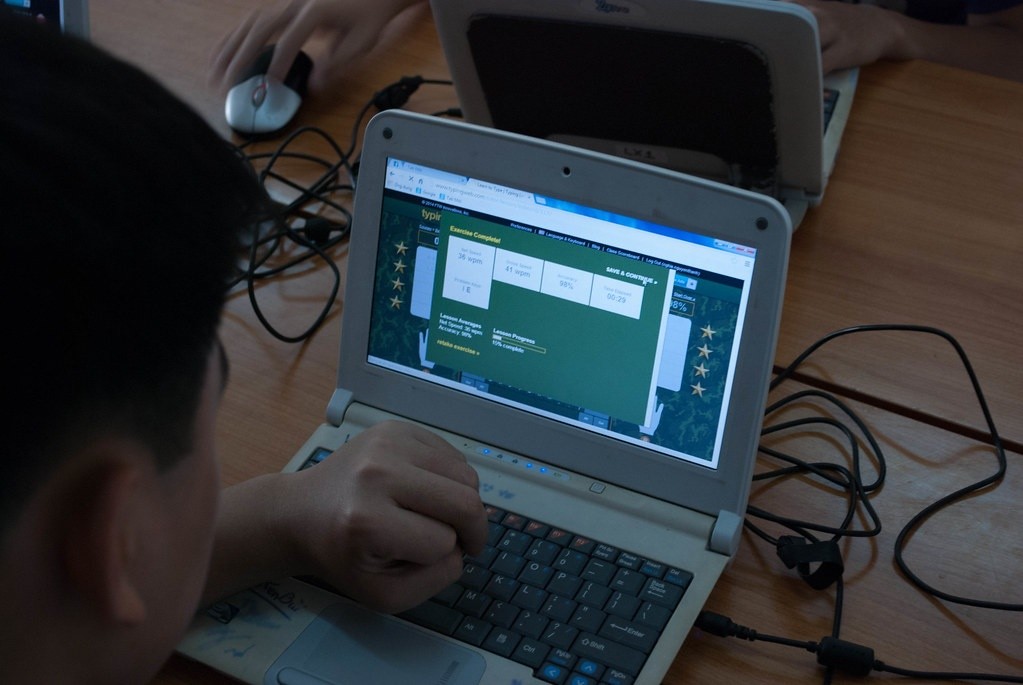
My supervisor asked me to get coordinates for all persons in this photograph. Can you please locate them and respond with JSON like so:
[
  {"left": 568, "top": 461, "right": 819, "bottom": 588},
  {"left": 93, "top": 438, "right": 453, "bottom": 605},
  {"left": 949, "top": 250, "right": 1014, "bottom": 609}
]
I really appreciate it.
[
  {"left": 0, "top": 6, "right": 488, "bottom": 685},
  {"left": 209, "top": 1, "right": 1023, "bottom": 93}
]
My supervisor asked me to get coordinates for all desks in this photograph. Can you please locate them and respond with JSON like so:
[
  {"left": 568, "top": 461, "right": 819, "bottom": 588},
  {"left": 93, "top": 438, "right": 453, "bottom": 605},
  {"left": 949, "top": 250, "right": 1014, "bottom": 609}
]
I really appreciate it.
[{"left": 88, "top": 0, "right": 1023, "bottom": 685}]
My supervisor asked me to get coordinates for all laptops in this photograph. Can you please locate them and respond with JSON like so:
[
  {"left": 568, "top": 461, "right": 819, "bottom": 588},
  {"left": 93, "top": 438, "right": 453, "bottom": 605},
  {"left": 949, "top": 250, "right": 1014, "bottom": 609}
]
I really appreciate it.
[
  {"left": 162, "top": 105, "right": 790, "bottom": 685},
  {"left": 430, "top": 0, "right": 859, "bottom": 207}
]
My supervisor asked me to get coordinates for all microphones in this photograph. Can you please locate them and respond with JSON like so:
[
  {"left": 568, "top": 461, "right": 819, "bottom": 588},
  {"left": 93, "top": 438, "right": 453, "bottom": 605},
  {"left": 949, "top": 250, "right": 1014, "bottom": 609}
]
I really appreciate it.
[{"left": 226, "top": 43, "right": 313, "bottom": 143}]
